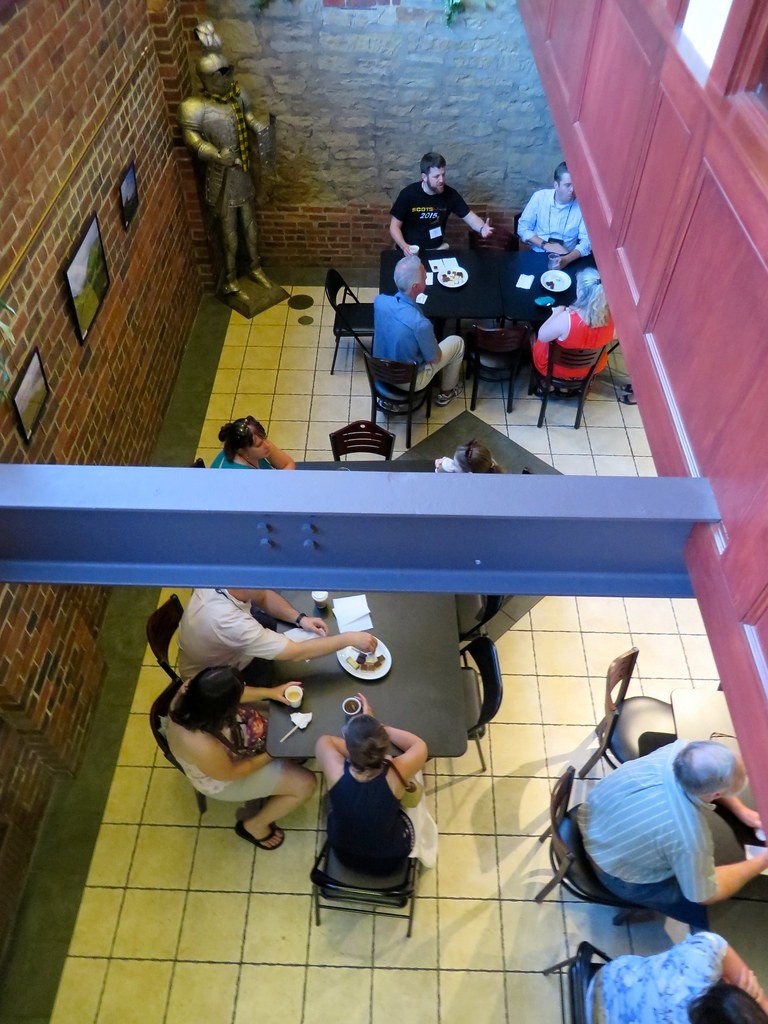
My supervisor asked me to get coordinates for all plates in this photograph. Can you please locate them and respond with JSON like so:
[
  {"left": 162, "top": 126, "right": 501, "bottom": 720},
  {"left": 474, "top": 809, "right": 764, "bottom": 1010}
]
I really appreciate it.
[
  {"left": 437, "top": 265, "right": 468, "bottom": 287},
  {"left": 540, "top": 268, "right": 572, "bottom": 292},
  {"left": 336, "top": 636, "right": 393, "bottom": 680}
]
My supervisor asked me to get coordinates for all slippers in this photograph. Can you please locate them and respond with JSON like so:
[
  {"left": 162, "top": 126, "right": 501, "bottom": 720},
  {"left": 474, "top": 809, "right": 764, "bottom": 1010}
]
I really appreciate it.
[
  {"left": 234, "top": 821, "right": 286, "bottom": 851},
  {"left": 617, "top": 384, "right": 637, "bottom": 405}
]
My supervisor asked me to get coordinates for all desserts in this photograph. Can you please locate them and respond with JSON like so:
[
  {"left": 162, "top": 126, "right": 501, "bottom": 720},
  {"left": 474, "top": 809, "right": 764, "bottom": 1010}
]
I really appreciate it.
[
  {"left": 442, "top": 270, "right": 463, "bottom": 284},
  {"left": 345, "top": 653, "right": 385, "bottom": 672}
]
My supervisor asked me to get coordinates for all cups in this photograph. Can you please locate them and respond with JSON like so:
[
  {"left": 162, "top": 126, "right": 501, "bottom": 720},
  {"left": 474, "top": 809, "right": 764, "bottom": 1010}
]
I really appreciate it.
[
  {"left": 311, "top": 590, "right": 328, "bottom": 609},
  {"left": 547, "top": 252, "right": 559, "bottom": 270},
  {"left": 342, "top": 697, "right": 361, "bottom": 716},
  {"left": 284, "top": 685, "right": 303, "bottom": 708}
]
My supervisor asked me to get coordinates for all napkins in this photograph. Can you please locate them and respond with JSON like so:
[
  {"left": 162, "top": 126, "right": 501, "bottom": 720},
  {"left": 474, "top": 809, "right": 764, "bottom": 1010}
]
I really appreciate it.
[
  {"left": 516, "top": 273, "right": 535, "bottom": 292},
  {"left": 332, "top": 590, "right": 374, "bottom": 634}
]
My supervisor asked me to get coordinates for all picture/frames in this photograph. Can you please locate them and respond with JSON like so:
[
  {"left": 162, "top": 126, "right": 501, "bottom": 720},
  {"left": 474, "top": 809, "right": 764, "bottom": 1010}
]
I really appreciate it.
[
  {"left": 59, "top": 211, "right": 111, "bottom": 346},
  {"left": 117, "top": 161, "right": 142, "bottom": 231},
  {"left": 8, "top": 348, "right": 51, "bottom": 443}
]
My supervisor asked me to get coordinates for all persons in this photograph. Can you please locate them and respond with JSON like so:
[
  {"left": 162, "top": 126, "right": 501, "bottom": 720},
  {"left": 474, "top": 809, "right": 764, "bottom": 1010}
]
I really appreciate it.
[
  {"left": 164, "top": 665, "right": 317, "bottom": 850},
  {"left": 388, "top": 151, "right": 495, "bottom": 257},
  {"left": 434, "top": 438, "right": 503, "bottom": 474},
  {"left": 314, "top": 693, "right": 428, "bottom": 875},
  {"left": 373, "top": 255, "right": 464, "bottom": 406},
  {"left": 531, "top": 269, "right": 615, "bottom": 398},
  {"left": 210, "top": 415, "right": 296, "bottom": 470},
  {"left": 584, "top": 931, "right": 768, "bottom": 1024},
  {"left": 517, "top": 162, "right": 592, "bottom": 270},
  {"left": 177, "top": 588, "right": 378, "bottom": 685},
  {"left": 179, "top": 21, "right": 275, "bottom": 297},
  {"left": 577, "top": 737, "right": 768, "bottom": 935}
]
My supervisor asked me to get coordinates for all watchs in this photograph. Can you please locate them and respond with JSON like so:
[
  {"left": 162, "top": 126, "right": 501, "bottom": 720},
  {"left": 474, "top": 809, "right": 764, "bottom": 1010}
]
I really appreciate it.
[{"left": 540, "top": 241, "right": 548, "bottom": 250}]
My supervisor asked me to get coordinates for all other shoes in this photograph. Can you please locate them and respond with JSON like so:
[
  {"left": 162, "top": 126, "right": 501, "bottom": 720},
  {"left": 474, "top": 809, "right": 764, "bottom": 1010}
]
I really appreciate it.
[{"left": 536, "top": 381, "right": 578, "bottom": 397}]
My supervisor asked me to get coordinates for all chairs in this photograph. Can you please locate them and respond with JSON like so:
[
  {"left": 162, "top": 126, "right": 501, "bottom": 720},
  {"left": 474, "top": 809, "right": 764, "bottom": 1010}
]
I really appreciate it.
[
  {"left": 149, "top": 678, "right": 209, "bottom": 814},
  {"left": 309, "top": 837, "right": 421, "bottom": 937},
  {"left": 329, "top": 421, "right": 396, "bottom": 463},
  {"left": 528, "top": 334, "right": 604, "bottom": 430},
  {"left": 534, "top": 767, "right": 647, "bottom": 928},
  {"left": 577, "top": 647, "right": 677, "bottom": 779},
  {"left": 455, "top": 590, "right": 506, "bottom": 642},
  {"left": 460, "top": 322, "right": 526, "bottom": 412},
  {"left": 512, "top": 211, "right": 530, "bottom": 254},
  {"left": 461, "top": 637, "right": 504, "bottom": 773},
  {"left": 361, "top": 352, "right": 432, "bottom": 449},
  {"left": 321, "top": 268, "right": 376, "bottom": 375},
  {"left": 145, "top": 595, "right": 187, "bottom": 681},
  {"left": 543, "top": 941, "right": 612, "bottom": 1023}
]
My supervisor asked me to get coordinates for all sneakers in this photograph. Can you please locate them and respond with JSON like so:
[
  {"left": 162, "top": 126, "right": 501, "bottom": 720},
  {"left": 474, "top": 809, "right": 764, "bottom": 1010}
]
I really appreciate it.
[
  {"left": 378, "top": 399, "right": 400, "bottom": 412},
  {"left": 435, "top": 380, "right": 465, "bottom": 407}
]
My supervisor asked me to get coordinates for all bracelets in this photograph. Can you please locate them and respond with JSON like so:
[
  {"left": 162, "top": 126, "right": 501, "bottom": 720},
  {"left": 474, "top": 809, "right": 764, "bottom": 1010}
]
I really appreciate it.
[
  {"left": 296, "top": 613, "right": 307, "bottom": 628},
  {"left": 738, "top": 963, "right": 763, "bottom": 1002},
  {"left": 480, "top": 226, "right": 483, "bottom": 235}
]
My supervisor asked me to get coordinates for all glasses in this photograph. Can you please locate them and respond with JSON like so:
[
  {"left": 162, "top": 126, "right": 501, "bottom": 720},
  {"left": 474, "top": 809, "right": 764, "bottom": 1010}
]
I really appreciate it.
[
  {"left": 465, "top": 438, "right": 477, "bottom": 472},
  {"left": 232, "top": 415, "right": 257, "bottom": 444}
]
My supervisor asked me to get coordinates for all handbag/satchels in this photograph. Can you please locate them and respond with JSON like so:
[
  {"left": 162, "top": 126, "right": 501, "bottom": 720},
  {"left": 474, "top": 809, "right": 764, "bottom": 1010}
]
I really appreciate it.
[
  {"left": 203, "top": 705, "right": 268, "bottom": 758},
  {"left": 384, "top": 758, "right": 422, "bottom": 808}
]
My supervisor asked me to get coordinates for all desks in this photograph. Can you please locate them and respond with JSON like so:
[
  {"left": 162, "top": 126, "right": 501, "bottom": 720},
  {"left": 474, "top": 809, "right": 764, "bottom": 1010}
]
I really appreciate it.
[
  {"left": 703, "top": 897, "right": 768, "bottom": 1017},
  {"left": 670, "top": 687, "right": 768, "bottom": 899},
  {"left": 508, "top": 247, "right": 593, "bottom": 320},
  {"left": 266, "top": 589, "right": 468, "bottom": 761},
  {"left": 378, "top": 246, "right": 505, "bottom": 339}
]
